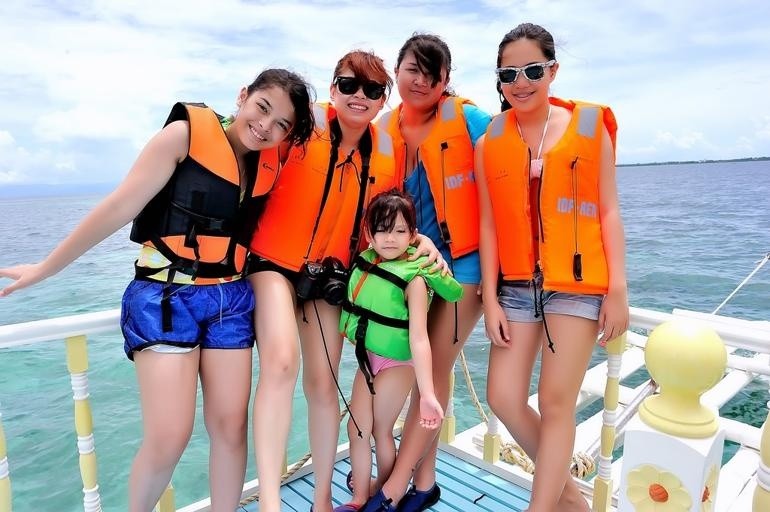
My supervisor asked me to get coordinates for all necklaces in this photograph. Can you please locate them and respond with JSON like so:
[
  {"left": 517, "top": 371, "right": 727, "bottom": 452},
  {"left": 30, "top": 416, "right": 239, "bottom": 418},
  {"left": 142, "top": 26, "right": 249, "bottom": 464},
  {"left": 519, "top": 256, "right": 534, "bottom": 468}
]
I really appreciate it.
[{"left": 514, "top": 105, "right": 553, "bottom": 160}]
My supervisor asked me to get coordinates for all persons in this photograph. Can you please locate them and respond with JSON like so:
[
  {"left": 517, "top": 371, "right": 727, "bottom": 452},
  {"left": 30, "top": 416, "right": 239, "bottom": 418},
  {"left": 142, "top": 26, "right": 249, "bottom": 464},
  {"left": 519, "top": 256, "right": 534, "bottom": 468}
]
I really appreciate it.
[
  {"left": 359, "top": 34, "right": 492, "bottom": 512},
  {"left": 474, "top": 22, "right": 629, "bottom": 512},
  {"left": 0, "top": 68, "right": 319, "bottom": 512},
  {"left": 332, "top": 188, "right": 447, "bottom": 511},
  {"left": 240, "top": 50, "right": 455, "bottom": 512}
]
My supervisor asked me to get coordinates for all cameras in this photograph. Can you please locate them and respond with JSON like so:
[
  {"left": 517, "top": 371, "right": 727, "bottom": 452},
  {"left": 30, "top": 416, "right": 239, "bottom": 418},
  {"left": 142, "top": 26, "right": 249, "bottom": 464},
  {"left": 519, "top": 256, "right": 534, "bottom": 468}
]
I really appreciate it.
[{"left": 296, "top": 255, "right": 351, "bottom": 306}]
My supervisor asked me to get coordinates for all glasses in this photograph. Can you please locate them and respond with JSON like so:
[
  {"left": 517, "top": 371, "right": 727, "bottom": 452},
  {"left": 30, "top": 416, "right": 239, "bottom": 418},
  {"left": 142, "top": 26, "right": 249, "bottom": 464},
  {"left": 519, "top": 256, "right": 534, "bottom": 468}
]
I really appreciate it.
[
  {"left": 333, "top": 76, "right": 386, "bottom": 100},
  {"left": 495, "top": 60, "right": 556, "bottom": 84}
]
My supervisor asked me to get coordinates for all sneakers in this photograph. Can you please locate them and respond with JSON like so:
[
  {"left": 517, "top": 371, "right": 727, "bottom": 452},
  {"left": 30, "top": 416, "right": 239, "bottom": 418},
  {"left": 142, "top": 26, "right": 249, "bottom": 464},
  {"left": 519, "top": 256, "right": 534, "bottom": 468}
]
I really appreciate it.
[{"left": 310, "top": 471, "right": 440, "bottom": 512}]
